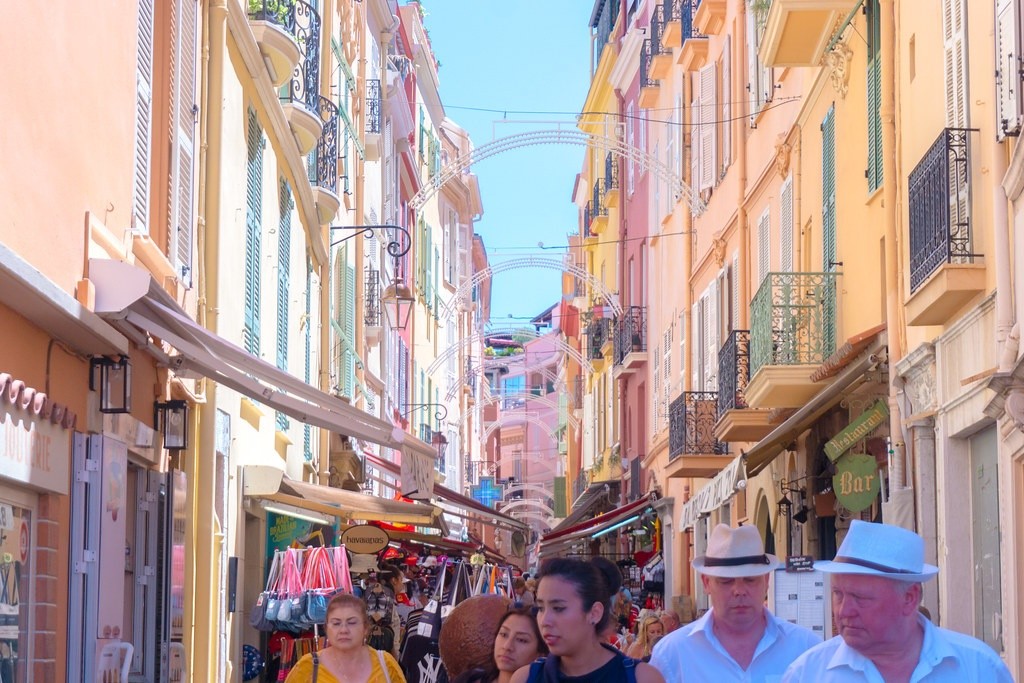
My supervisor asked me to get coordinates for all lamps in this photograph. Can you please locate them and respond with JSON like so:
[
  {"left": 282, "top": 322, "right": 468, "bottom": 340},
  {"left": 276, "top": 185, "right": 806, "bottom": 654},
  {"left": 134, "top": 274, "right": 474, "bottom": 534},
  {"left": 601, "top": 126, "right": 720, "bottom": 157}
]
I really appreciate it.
[
  {"left": 328, "top": 224, "right": 416, "bottom": 333},
  {"left": 401, "top": 403, "right": 449, "bottom": 457}
]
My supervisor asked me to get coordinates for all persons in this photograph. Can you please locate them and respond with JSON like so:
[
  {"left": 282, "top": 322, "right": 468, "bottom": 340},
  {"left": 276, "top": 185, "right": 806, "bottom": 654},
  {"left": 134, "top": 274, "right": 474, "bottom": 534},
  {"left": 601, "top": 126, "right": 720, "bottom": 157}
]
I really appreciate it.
[
  {"left": 508, "top": 555, "right": 665, "bottom": 683},
  {"left": 283, "top": 593, "right": 407, "bottom": 683},
  {"left": 448, "top": 608, "right": 550, "bottom": 683},
  {"left": 375, "top": 561, "right": 686, "bottom": 663},
  {"left": 779, "top": 520, "right": 1014, "bottom": 683},
  {"left": 651, "top": 523, "right": 824, "bottom": 683}
]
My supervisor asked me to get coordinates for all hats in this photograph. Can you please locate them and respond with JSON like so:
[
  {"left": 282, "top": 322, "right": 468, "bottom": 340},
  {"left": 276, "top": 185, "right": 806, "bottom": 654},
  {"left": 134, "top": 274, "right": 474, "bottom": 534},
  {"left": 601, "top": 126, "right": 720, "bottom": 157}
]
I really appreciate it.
[
  {"left": 813, "top": 518, "right": 938, "bottom": 581},
  {"left": 692, "top": 523, "right": 779, "bottom": 576},
  {"left": 381, "top": 546, "right": 452, "bottom": 584}
]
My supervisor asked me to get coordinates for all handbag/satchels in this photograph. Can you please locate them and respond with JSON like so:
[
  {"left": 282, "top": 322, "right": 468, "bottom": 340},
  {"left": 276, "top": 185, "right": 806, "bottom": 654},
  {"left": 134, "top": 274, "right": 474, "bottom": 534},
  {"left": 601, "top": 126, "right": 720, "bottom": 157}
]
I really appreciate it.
[
  {"left": 250, "top": 543, "right": 356, "bottom": 681},
  {"left": 417, "top": 557, "right": 516, "bottom": 643}
]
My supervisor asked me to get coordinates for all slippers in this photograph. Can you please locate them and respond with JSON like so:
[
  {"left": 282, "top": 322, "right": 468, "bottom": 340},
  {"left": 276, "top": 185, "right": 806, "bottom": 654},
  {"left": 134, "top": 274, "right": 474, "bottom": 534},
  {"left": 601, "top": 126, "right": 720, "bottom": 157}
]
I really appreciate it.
[
  {"left": 364, "top": 585, "right": 378, "bottom": 624},
  {"left": 366, "top": 624, "right": 394, "bottom": 652},
  {"left": 378, "top": 585, "right": 394, "bottom": 624}
]
